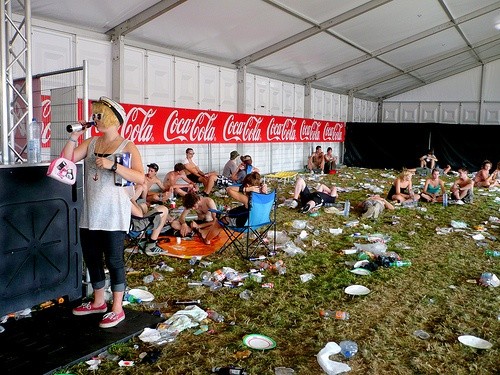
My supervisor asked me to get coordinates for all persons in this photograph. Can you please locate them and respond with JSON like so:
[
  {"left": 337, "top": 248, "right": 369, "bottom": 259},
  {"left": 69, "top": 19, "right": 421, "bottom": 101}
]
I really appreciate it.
[
  {"left": 324, "top": 147, "right": 337, "bottom": 174},
  {"left": 288, "top": 176, "right": 338, "bottom": 213},
  {"left": 126, "top": 161, "right": 201, "bottom": 202},
  {"left": 420, "top": 168, "right": 449, "bottom": 204},
  {"left": 123, "top": 193, "right": 170, "bottom": 256},
  {"left": 170, "top": 193, "right": 217, "bottom": 238},
  {"left": 358, "top": 195, "right": 394, "bottom": 219},
  {"left": 184, "top": 148, "right": 223, "bottom": 197},
  {"left": 309, "top": 146, "right": 325, "bottom": 175},
  {"left": 223, "top": 151, "right": 241, "bottom": 179},
  {"left": 450, "top": 167, "right": 474, "bottom": 205},
  {"left": 472, "top": 160, "right": 500, "bottom": 188},
  {"left": 198, "top": 154, "right": 260, "bottom": 197},
  {"left": 196, "top": 170, "right": 268, "bottom": 244},
  {"left": 60, "top": 95, "right": 146, "bottom": 328},
  {"left": 406, "top": 164, "right": 455, "bottom": 175},
  {"left": 387, "top": 170, "right": 420, "bottom": 205},
  {"left": 419, "top": 150, "right": 438, "bottom": 169}
]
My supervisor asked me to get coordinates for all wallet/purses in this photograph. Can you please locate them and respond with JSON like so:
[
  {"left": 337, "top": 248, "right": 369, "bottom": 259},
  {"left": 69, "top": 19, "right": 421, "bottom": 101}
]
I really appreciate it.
[{"left": 46, "top": 150, "right": 77, "bottom": 185}]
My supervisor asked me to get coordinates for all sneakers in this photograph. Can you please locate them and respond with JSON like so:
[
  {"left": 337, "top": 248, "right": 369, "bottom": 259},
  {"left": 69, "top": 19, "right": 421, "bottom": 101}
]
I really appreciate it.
[
  {"left": 99, "top": 311, "right": 125, "bottom": 328},
  {"left": 72, "top": 302, "right": 107, "bottom": 315}
]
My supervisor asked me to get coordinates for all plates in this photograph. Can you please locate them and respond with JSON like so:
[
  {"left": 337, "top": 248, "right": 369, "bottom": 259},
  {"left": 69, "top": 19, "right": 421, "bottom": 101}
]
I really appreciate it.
[
  {"left": 458, "top": 335, "right": 493, "bottom": 349},
  {"left": 128, "top": 289, "right": 154, "bottom": 301},
  {"left": 345, "top": 286, "right": 371, "bottom": 295},
  {"left": 242, "top": 333, "right": 278, "bottom": 349},
  {"left": 350, "top": 268, "right": 373, "bottom": 276}
]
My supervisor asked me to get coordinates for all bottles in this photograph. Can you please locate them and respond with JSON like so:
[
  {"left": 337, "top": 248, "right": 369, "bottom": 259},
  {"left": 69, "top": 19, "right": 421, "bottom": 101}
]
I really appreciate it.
[
  {"left": 344, "top": 199, "right": 352, "bottom": 218},
  {"left": 389, "top": 260, "right": 412, "bottom": 267},
  {"left": 325, "top": 202, "right": 342, "bottom": 208},
  {"left": 443, "top": 193, "right": 448, "bottom": 206},
  {"left": 122, "top": 294, "right": 141, "bottom": 304},
  {"left": 309, "top": 213, "right": 319, "bottom": 217},
  {"left": 183, "top": 268, "right": 195, "bottom": 279},
  {"left": 485, "top": 250, "right": 500, "bottom": 258},
  {"left": 319, "top": 310, "right": 350, "bottom": 320},
  {"left": 66, "top": 122, "right": 95, "bottom": 133},
  {"left": 171, "top": 300, "right": 201, "bottom": 307},
  {"left": 212, "top": 367, "right": 246, "bottom": 374},
  {"left": 340, "top": 341, "right": 359, "bottom": 358},
  {"left": 26, "top": 118, "right": 41, "bottom": 165},
  {"left": 204, "top": 309, "right": 227, "bottom": 322}
]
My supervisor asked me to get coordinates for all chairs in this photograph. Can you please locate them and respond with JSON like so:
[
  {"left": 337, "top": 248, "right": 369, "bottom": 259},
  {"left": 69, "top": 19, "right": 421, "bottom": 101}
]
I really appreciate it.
[
  {"left": 122, "top": 212, "right": 163, "bottom": 267},
  {"left": 207, "top": 188, "right": 278, "bottom": 262}
]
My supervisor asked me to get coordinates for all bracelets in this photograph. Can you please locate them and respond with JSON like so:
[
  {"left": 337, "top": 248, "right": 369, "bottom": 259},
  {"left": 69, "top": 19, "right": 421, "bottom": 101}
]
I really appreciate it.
[
  {"left": 69, "top": 136, "right": 78, "bottom": 143},
  {"left": 239, "top": 185, "right": 247, "bottom": 195}
]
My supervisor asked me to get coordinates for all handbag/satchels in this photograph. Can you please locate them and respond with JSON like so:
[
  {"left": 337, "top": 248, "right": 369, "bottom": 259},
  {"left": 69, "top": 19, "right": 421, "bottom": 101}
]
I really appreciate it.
[{"left": 419, "top": 192, "right": 433, "bottom": 203}]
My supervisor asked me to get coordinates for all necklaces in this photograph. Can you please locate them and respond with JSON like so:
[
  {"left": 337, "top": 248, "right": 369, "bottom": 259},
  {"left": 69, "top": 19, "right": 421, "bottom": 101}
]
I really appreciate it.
[{"left": 93, "top": 135, "right": 120, "bottom": 181}]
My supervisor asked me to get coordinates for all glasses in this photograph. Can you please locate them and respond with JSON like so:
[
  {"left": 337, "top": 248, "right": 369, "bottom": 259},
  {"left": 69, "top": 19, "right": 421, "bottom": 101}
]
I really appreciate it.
[{"left": 91, "top": 113, "right": 103, "bottom": 119}]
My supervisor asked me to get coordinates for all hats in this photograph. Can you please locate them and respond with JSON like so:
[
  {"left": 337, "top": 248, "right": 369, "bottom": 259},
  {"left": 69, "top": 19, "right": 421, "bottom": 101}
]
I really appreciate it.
[
  {"left": 98, "top": 97, "right": 127, "bottom": 125},
  {"left": 147, "top": 163, "right": 159, "bottom": 172},
  {"left": 230, "top": 151, "right": 240, "bottom": 159},
  {"left": 241, "top": 155, "right": 252, "bottom": 164}
]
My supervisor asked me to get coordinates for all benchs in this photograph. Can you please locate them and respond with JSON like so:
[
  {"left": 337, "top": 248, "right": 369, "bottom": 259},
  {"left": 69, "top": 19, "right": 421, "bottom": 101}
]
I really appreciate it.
[{"left": 264, "top": 171, "right": 298, "bottom": 185}]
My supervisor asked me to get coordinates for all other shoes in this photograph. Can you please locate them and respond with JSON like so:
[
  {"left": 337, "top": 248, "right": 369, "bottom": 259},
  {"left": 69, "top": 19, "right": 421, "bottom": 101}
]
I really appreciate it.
[
  {"left": 145, "top": 246, "right": 165, "bottom": 255},
  {"left": 449, "top": 199, "right": 464, "bottom": 205},
  {"left": 303, "top": 204, "right": 311, "bottom": 213},
  {"left": 289, "top": 200, "right": 298, "bottom": 210}
]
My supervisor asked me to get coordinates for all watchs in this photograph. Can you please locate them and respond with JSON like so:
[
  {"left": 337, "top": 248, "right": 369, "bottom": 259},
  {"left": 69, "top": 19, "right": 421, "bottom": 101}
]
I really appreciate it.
[{"left": 110, "top": 162, "right": 117, "bottom": 174}]
